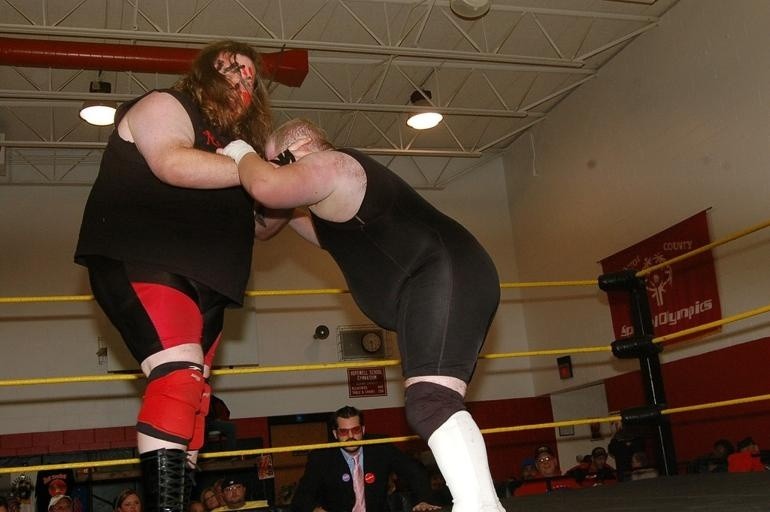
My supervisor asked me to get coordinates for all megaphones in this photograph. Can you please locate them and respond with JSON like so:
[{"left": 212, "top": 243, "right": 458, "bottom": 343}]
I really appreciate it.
[{"left": 313, "top": 325, "right": 329, "bottom": 340}]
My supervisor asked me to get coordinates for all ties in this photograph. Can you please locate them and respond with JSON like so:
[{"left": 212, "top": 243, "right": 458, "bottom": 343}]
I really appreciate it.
[{"left": 352, "top": 454, "right": 367, "bottom": 512}]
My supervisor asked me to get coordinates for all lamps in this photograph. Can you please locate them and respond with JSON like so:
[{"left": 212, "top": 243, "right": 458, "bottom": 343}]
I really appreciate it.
[
  {"left": 78, "top": 66, "right": 121, "bottom": 128},
  {"left": 405, "top": 89, "right": 443, "bottom": 131}
]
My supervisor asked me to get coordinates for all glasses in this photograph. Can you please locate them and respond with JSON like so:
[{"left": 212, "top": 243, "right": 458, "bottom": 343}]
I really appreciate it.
[
  {"left": 338, "top": 426, "right": 362, "bottom": 436},
  {"left": 537, "top": 457, "right": 552, "bottom": 463},
  {"left": 225, "top": 486, "right": 242, "bottom": 491}
]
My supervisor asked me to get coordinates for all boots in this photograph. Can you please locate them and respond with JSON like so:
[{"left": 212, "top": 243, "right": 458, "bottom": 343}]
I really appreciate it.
[
  {"left": 427, "top": 410, "right": 507, "bottom": 512},
  {"left": 137, "top": 447, "right": 199, "bottom": 512}
]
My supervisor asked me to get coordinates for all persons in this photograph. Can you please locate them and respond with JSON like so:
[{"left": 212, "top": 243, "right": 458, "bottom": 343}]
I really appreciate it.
[
  {"left": 689, "top": 435, "right": 769, "bottom": 478},
  {"left": 1, "top": 472, "right": 71, "bottom": 510},
  {"left": 200, "top": 478, "right": 270, "bottom": 511},
  {"left": 509, "top": 443, "right": 658, "bottom": 494},
  {"left": 115, "top": 491, "right": 142, "bottom": 511},
  {"left": 386, "top": 466, "right": 452, "bottom": 510},
  {"left": 214, "top": 115, "right": 508, "bottom": 510},
  {"left": 72, "top": 38, "right": 315, "bottom": 510},
  {"left": 288, "top": 406, "right": 435, "bottom": 510}
]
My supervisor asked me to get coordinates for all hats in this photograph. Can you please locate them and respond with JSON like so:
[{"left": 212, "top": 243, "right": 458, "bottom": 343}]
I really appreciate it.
[
  {"left": 48, "top": 494, "right": 73, "bottom": 511},
  {"left": 221, "top": 478, "right": 243, "bottom": 490},
  {"left": 522, "top": 459, "right": 535, "bottom": 466},
  {"left": 534, "top": 446, "right": 556, "bottom": 458},
  {"left": 592, "top": 447, "right": 608, "bottom": 459}
]
